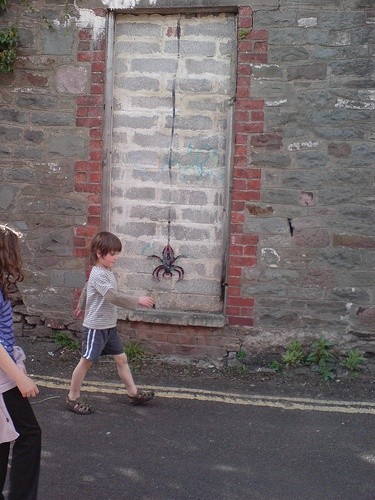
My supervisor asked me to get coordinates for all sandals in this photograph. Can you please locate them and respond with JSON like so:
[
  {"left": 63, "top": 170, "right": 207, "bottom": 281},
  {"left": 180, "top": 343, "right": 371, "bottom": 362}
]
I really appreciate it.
[
  {"left": 64, "top": 395, "right": 97, "bottom": 415},
  {"left": 127, "top": 389, "right": 154, "bottom": 404}
]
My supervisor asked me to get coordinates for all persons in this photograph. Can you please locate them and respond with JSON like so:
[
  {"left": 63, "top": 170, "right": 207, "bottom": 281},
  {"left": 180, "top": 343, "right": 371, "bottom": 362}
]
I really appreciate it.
[
  {"left": 63, "top": 232, "right": 155, "bottom": 414},
  {"left": 0, "top": 225, "right": 42, "bottom": 500}
]
